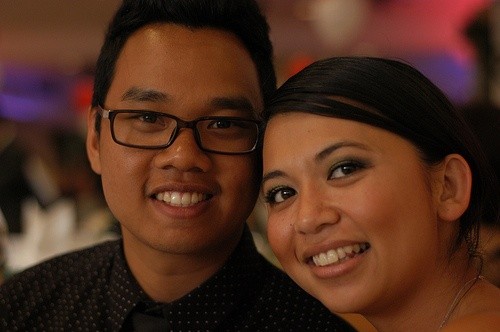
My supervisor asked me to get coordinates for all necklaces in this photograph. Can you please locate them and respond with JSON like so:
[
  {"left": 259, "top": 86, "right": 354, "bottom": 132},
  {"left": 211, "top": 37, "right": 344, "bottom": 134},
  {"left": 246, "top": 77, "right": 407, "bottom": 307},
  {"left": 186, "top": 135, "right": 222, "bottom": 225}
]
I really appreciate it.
[{"left": 441, "top": 274, "right": 486, "bottom": 329}]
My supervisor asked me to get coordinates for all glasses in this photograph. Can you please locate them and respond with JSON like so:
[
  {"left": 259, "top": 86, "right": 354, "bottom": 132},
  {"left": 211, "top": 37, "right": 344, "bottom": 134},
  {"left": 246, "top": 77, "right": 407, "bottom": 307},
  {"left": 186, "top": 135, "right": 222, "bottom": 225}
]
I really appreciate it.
[{"left": 97, "top": 103, "right": 266, "bottom": 155}]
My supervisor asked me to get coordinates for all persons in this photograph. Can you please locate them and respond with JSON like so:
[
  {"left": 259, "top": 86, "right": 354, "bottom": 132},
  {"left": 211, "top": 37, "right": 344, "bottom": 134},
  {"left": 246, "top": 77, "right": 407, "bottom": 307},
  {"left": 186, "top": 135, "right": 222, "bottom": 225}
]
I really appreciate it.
[
  {"left": 1, "top": 0, "right": 356, "bottom": 332},
  {"left": 261, "top": 56, "right": 500, "bottom": 332}
]
